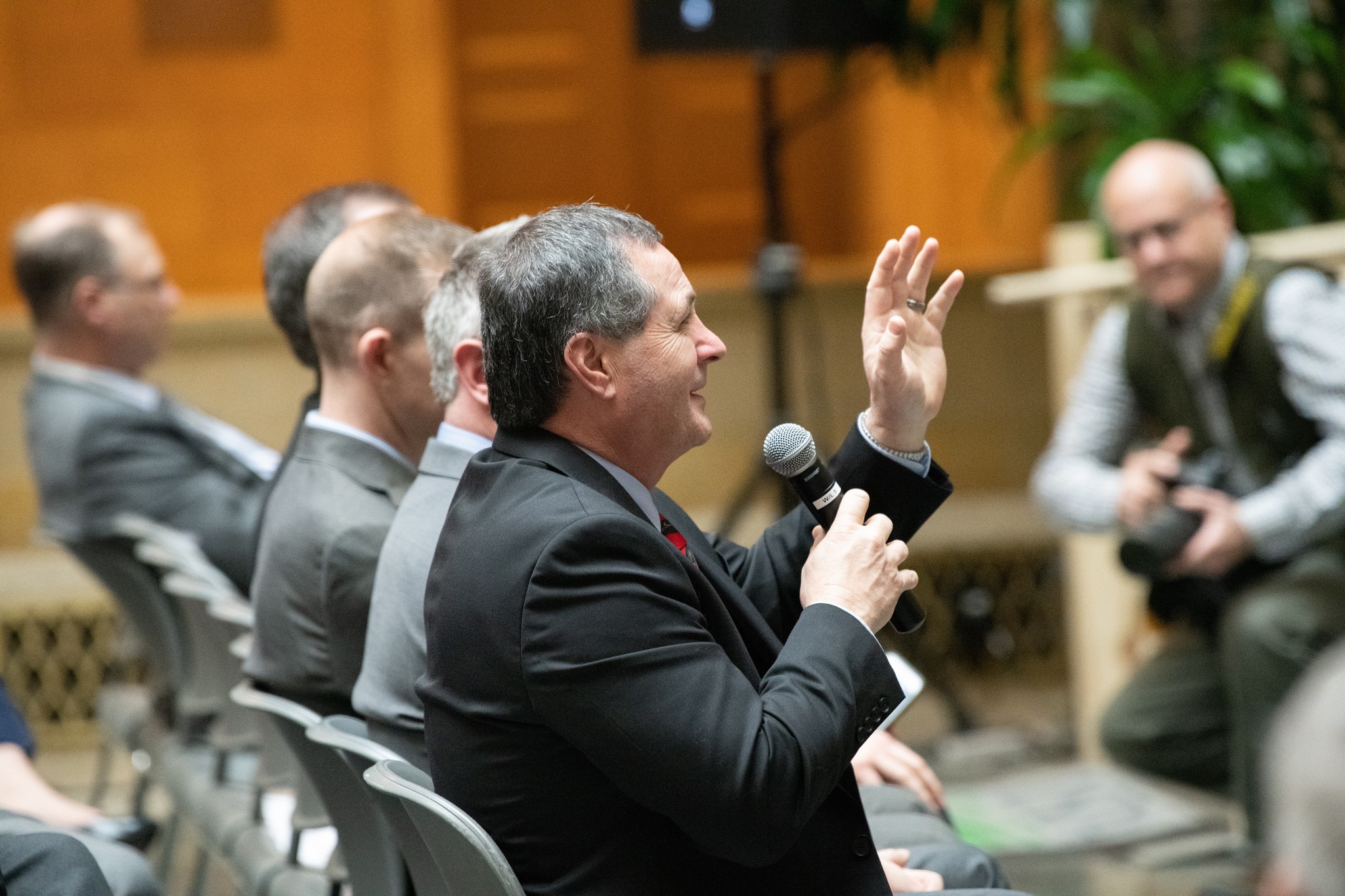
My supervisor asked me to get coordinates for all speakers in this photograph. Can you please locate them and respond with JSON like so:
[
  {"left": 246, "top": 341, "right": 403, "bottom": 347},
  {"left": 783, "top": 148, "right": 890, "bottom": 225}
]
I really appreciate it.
[{"left": 630, "top": 1, "right": 909, "bottom": 50}]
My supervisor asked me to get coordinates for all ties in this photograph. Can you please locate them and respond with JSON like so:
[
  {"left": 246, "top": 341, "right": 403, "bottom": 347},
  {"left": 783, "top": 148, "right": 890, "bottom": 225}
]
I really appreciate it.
[{"left": 658, "top": 510, "right": 686, "bottom": 557}]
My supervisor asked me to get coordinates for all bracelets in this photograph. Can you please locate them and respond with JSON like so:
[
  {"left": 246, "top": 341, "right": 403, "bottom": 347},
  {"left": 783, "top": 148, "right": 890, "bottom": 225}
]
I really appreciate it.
[{"left": 862, "top": 412, "right": 927, "bottom": 464}]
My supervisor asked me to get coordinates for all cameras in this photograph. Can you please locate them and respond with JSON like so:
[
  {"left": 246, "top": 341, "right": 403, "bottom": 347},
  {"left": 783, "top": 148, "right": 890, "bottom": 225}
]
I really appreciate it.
[{"left": 1116, "top": 447, "right": 1240, "bottom": 575}]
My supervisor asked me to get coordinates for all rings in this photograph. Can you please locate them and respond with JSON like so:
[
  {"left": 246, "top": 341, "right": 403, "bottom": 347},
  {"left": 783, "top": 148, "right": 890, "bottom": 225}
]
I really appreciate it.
[{"left": 906, "top": 298, "right": 925, "bottom": 311}]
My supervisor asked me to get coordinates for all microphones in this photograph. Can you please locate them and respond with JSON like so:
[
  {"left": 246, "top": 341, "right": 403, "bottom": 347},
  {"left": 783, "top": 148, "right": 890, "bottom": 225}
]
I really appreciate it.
[{"left": 764, "top": 423, "right": 926, "bottom": 634}]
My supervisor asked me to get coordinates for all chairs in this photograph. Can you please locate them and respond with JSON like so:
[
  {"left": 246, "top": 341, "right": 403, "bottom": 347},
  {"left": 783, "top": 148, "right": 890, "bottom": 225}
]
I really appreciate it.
[{"left": 32, "top": 510, "right": 527, "bottom": 896}]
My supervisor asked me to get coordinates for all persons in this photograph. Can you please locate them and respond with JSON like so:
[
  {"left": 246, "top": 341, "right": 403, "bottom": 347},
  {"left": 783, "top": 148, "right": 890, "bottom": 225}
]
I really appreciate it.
[
  {"left": 351, "top": 214, "right": 1014, "bottom": 893},
  {"left": 1, "top": 686, "right": 162, "bottom": 896},
  {"left": 415, "top": 200, "right": 1030, "bottom": 896},
  {"left": 14, "top": 204, "right": 284, "bottom": 602},
  {"left": 253, "top": 180, "right": 433, "bottom": 585},
  {"left": 1025, "top": 139, "right": 1344, "bottom": 892},
  {"left": 247, "top": 218, "right": 971, "bottom": 850}
]
